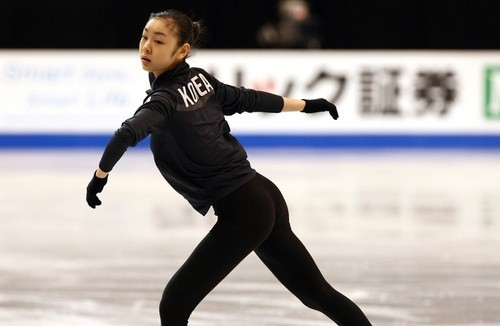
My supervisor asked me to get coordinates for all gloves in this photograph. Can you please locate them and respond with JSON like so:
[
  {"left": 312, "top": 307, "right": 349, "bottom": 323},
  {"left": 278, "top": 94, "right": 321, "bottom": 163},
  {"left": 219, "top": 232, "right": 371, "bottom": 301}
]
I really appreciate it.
[
  {"left": 300, "top": 98, "right": 340, "bottom": 120},
  {"left": 86, "top": 170, "right": 109, "bottom": 209}
]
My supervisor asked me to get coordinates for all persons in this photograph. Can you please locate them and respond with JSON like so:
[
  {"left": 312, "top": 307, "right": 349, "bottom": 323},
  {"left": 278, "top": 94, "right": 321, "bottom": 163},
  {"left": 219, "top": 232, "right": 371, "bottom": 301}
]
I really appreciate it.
[
  {"left": 257, "top": 0, "right": 322, "bottom": 50},
  {"left": 86, "top": 11, "right": 373, "bottom": 326}
]
